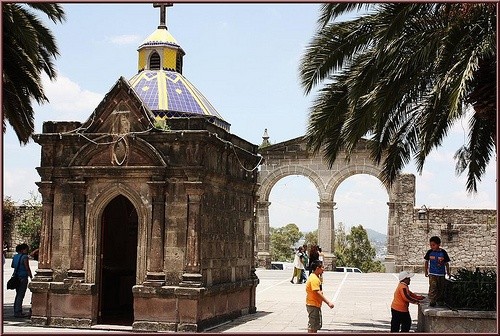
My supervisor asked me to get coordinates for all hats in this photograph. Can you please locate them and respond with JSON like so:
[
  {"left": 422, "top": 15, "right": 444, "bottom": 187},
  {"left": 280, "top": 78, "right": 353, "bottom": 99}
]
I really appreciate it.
[
  {"left": 298, "top": 247, "right": 303, "bottom": 250},
  {"left": 398, "top": 271, "right": 416, "bottom": 281}
]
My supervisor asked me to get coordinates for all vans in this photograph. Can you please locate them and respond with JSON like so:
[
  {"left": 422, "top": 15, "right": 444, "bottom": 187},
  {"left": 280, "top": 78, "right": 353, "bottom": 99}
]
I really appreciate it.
[{"left": 335, "top": 266, "right": 363, "bottom": 273}]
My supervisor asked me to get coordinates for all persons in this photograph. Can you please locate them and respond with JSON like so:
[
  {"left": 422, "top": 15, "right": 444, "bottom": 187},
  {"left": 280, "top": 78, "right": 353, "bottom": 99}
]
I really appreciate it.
[
  {"left": 424, "top": 236, "right": 451, "bottom": 307},
  {"left": 390, "top": 271, "right": 425, "bottom": 332},
  {"left": 305, "top": 260, "right": 334, "bottom": 333},
  {"left": 290, "top": 244, "right": 324, "bottom": 284},
  {"left": 11, "top": 244, "right": 33, "bottom": 318}
]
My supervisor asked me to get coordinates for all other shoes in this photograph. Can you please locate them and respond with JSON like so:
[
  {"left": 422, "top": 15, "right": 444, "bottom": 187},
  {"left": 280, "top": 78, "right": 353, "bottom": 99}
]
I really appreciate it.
[
  {"left": 303, "top": 279, "right": 307, "bottom": 283},
  {"left": 296, "top": 282, "right": 302, "bottom": 284},
  {"left": 290, "top": 280, "right": 294, "bottom": 284},
  {"left": 299, "top": 279, "right": 302, "bottom": 282},
  {"left": 438, "top": 302, "right": 444, "bottom": 306},
  {"left": 430, "top": 302, "right": 436, "bottom": 307},
  {"left": 13, "top": 312, "right": 28, "bottom": 318}
]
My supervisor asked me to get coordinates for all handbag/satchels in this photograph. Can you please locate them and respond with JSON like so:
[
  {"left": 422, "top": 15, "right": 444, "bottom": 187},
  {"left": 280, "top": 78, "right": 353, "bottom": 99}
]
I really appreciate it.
[{"left": 7, "top": 275, "right": 18, "bottom": 290}]
